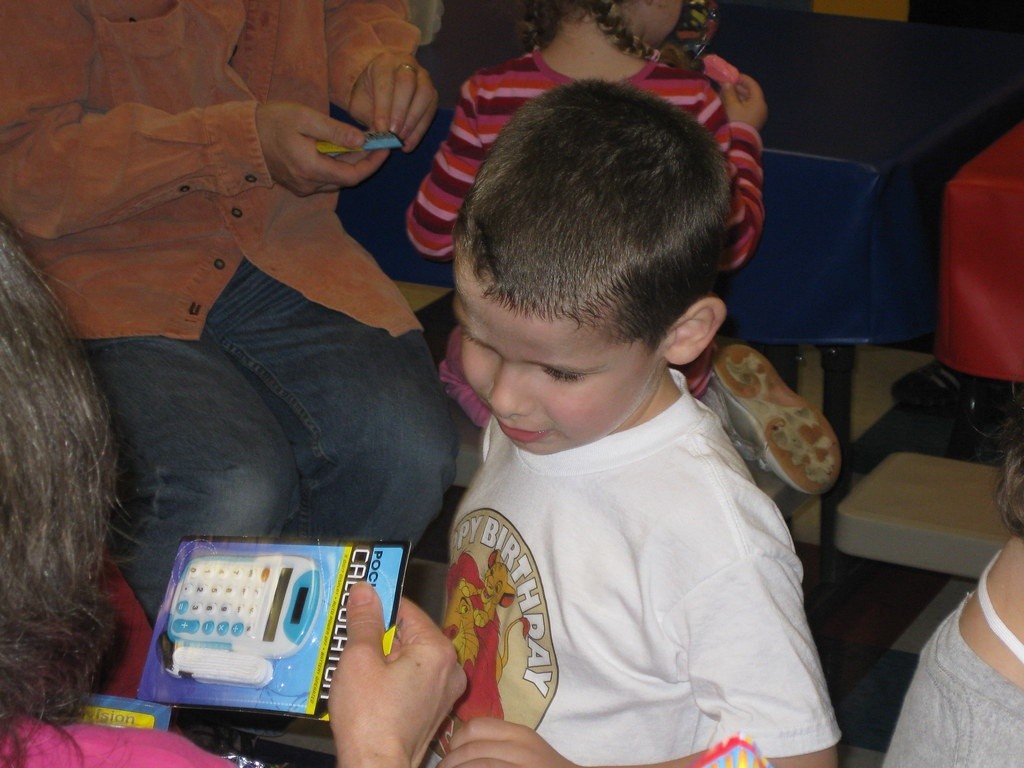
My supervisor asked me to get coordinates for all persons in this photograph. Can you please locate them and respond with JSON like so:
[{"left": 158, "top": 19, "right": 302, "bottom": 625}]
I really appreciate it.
[
  {"left": 414, "top": 75, "right": 845, "bottom": 768},
  {"left": 0, "top": 212, "right": 469, "bottom": 768},
  {"left": 405, "top": 0, "right": 846, "bottom": 497},
  {"left": 882, "top": 388, "right": 1024, "bottom": 768},
  {"left": 0, "top": 0, "right": 460, "bottom": 735}
]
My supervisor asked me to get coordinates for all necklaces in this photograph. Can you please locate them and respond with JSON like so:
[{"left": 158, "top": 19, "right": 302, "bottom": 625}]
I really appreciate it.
[{"left": 977, "top": 549, "right": 1024, "bottom": 658}]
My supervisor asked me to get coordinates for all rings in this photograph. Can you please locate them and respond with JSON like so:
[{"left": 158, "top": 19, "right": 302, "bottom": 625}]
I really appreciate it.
[{"left": 397, "top": 62, "right": 418, "bottom": 72}]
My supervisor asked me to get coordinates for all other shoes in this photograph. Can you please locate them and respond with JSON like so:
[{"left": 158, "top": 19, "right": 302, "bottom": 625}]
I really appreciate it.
[
  {"left": 892, "top": 351, "right": 969, "bottom": 410},
  {"left": 714, "top": 344, "right": 842, "bottom": 496}
]
